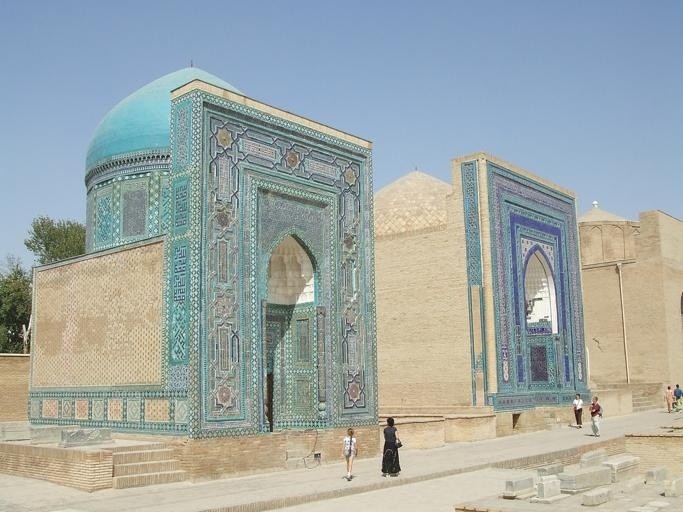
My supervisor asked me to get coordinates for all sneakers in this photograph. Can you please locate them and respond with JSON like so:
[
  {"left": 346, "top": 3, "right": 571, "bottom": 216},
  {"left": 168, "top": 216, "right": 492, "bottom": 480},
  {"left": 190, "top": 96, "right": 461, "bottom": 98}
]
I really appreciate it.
[
  {"left": 577, "top": 424, "right": 583, "bottom": 429},
  {"left": 592, "top": 432, "right": 600, "bottom": 436},
  {"left": 346, "top": 474, "right": 353, "bottom": 481}
]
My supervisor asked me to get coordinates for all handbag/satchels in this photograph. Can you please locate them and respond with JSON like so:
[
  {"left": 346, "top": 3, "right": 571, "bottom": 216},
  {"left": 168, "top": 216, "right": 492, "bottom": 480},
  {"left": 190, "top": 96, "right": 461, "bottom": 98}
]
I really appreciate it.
[
  {"left": 595, "top": 401, "right": 603, "bottom": 416},
  {"left": 395, "top": 443, "right": 403, "bottom": 448}
]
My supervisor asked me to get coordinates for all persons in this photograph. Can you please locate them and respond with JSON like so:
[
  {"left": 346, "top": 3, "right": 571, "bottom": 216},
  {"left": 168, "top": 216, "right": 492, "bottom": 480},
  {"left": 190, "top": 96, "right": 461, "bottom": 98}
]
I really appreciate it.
[
  {"left": 381, "top": 417, "right": 401, "bottom": 477},
  {"left": 674, "top": 384, "right": 683, "bottom": 400},
  {"left": 588, "top": 397, "right": 601, "bottom": 440},
  {"left": 664, "top": 385, "right": 674, "bottom": 413},
  {"left": 573, "top": 394, "right": 584, "bottom": 429},
  {"left": 342, "top": 429, "right": 358, "bottom": 481}
]
